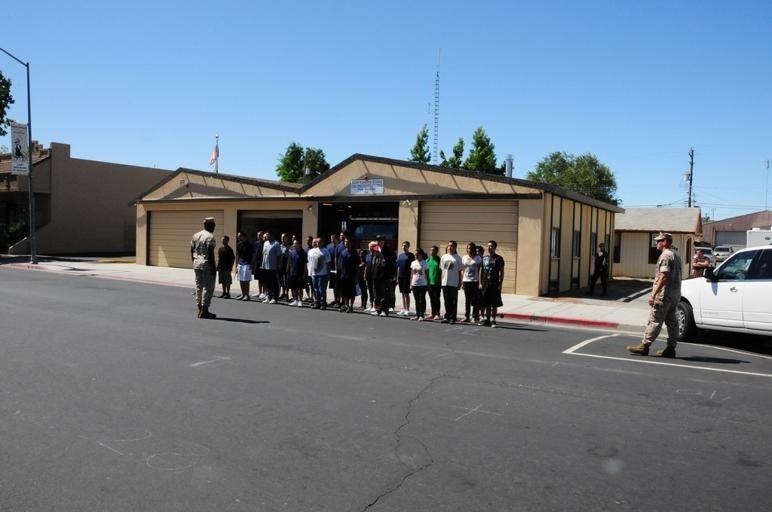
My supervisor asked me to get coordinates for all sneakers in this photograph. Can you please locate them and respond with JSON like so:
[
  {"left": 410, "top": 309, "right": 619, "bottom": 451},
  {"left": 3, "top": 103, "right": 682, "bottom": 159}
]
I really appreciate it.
[
  {"left": 439, "top": 317, "right": 449, "bottom": 325},
  {"left": 319, "top": 303, "right": 327, "bottom": 309},
  {"left": 261, "top": 297, "right": 269, "bottom": 303},
  {"left": 269, "top": 297, "right": 277, "bottom": 304},
  {"left": 370, "top": 306, "right": 376, "bottom": 311},
  {"left": 370, "top": 310, "right": 378, "bottom": 316},
  {"left": 346, "top": 305, "right": 352, "bottom": 313},
  {"left": 654, "top": 342, "right": 675, "bottom": 357},
  {"left": 332, "top": 302, "right": 339, "bottom": 307},
  {"left": 195, "top": 301, "right": 217, "bottom": 319},
  {"left": 288, "top": 299, "right": 298, "bottom": 307},
  {"left": 458, "top": 314, "right": 468, "bottom": 322},
  {"left": 396, "top": 308, "right": 404, "bottom": 317},
  {"left": 379, "top": 310, "right": 386, "bottom": 316},
  {"left": 470, "top": 315, "right": 478, "bottom": 323},
  {"left": 409, "top": 315, "right": 418, "bottom": 321},
  {"left": 217, "top": 292, "right": 226, "bottom": 298},
  {"left": 624, "top": 341, "right": 647, "bottom": 357},
  {"left": 235, "top": 294, "right": 243, "bottom": 300},
  {"left": 489, "top": 319, "right": 496, "bottom": 329},
  {"left": 339, "top": 304, "right": 345, "bottom": 313},
  {"left": 363, "top": 307, "right": 371, "bottom": 312},
  {"left": 310, "top": 302, "right": 319, "bottom": 310},
  {"left": 403, "top": 309, "right": 409, "bottom": 316},
  {"left": 433, "top": 314, "right": 441, "bottom": 321},
  {"left": 223, "top": 293, "right": 231, "bottom": 299},
  {"left": 298, "top": 300, "right": 303, "bottom": 307},
  {"left": 417, "top": 315, "right": 425, "bottom": 321},
  {"left": 449, "top": 317, "right": 455, "bottom": 323},
  {"left": 424, "top": 312, "right": 433, "bottom": 318},
  {"left": 242, "top": 295, "right": 250, "bottom": 301},
  {"left": 251, "top": 291, "right": 260, "bottom": 296},
  {"left": 389, "top": 307, "right": 394, "bottom": 314}
]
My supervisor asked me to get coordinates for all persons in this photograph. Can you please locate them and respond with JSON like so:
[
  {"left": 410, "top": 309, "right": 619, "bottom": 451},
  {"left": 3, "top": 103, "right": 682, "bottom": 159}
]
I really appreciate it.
[
  {"left": 477, "top": 240, "right": 505, "bottom": 328},
  {"left": 462, "top": 242, "right": 482, "bottom": 322},
  {"left": 439, "top": 240, "right": 462, "bottom": 323},
  {"left": 279, "top": 233, "right": 290, "bottom": 301},
  {"left": 692, "top": 251, "right": 712, "bottom": 280},
  {"left": 190, "top": 217, "right": 217, "bottom": 320},
  {"left": 626, "top": 232, "right": 683, "bottom": 358},
  {"left": 425, "top": 245, "right": 442, "bottom": 320},
  {"left": 289, "top": 240, "right": 307, "bottom": 307},
  {"left": 377, "top": 235, "right": 388, "bottom": 263},
  {"left": 304, "top": 236, "right": 314, "bottom": 300},
  {"left": 338, "top": 238, "right": 360, "bottom": 313},
  {"left": 364, "top": 242, "right": 377, "bottom": 312},
  {"left": 359, "top": 249, "right": 368, "bottom": 310},
  {"left": 389, "top": 251, "right": 398, "bottom": 312},
  {"left": 476, "top": 246, "right": 486, "bottom": 319},
  {"left": 307, "top": 239, "right": 321, "bottom": 302},
  {"left": 236, "top": 233, "right": 252, "bottom": 301},
  {"left": 217, "top": 236, "right": 236, "bottom": 299},
  {"left": 326, "top": 234, "right": 341, "bottom": 307},
  {"left": 409, "top": 248, "right": 429, "bottom": 321},
  {"left": 311, "top": 238, "right": 331, "bottom": 310},
  {"left": 336, "top": 232, "right": 346, "bottom": 306},
  {"left": 253, "top": 231, "right": 265, "bottom": 299},
  {"left": 590, "top": 243, "right": 610, "bottom": 298},
  {"left": 262, "top": 233, "right": 280, "bottom": 304},
  {"left": 370, "top": 245, "right": 389, "bottom": 316},
  {"left": 397, "top": 240, "right": 415, "bottom": 315}
]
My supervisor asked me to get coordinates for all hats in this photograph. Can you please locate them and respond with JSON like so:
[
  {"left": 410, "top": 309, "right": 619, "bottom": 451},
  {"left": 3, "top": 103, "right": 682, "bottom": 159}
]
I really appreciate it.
[
  {"left": 653, "top": 230, "right": 673, "bottom": 246},
  {"left": 598, "top": 241, "right": 606, "bottom": 246},
  {"left": 374, "top": 232, "right": 386, "bottom": 240},
  {"left": 202, "top": 216, "right": 217, "bottom": 224}
]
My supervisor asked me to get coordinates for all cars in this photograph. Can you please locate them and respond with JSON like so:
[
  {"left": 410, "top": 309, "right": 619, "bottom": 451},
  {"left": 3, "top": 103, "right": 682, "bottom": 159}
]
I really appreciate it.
[
  {"left": 713, "top": 245, "right": 734, "bottom": 261},
  {"left": 694, "top": 246, "right": 715, "bottom": 262}
]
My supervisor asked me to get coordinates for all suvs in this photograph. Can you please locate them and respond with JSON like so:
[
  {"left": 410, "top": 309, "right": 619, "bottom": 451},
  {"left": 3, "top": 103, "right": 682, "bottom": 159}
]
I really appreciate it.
[{"left": 675, "top": 245, "right": 771, "bottom": 339}]
